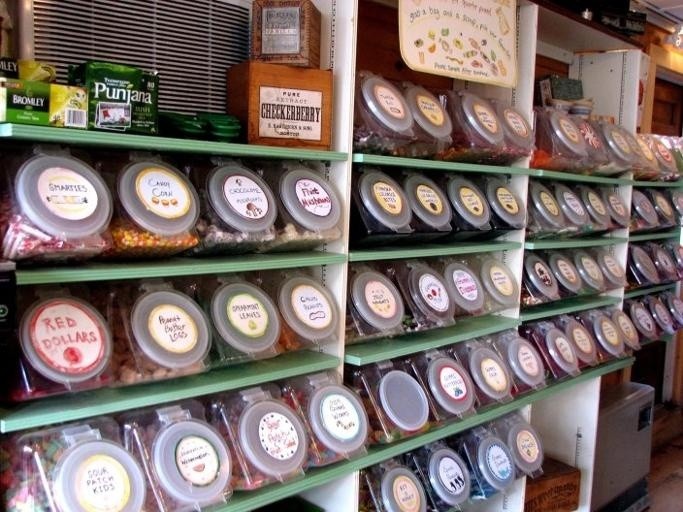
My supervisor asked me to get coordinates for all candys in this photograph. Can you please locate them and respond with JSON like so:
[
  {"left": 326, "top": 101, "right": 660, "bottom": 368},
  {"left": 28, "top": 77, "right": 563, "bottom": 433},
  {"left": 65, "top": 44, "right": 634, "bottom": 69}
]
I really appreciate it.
[{"left": 1, "top": 131, "right": 683, "bottom": 512}]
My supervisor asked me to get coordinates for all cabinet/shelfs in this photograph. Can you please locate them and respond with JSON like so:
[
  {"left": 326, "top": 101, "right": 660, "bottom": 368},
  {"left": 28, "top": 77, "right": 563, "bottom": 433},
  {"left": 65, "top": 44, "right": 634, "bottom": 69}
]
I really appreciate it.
[{"left": 0, "top": 0, "right": 683, "bottom": 511}]
